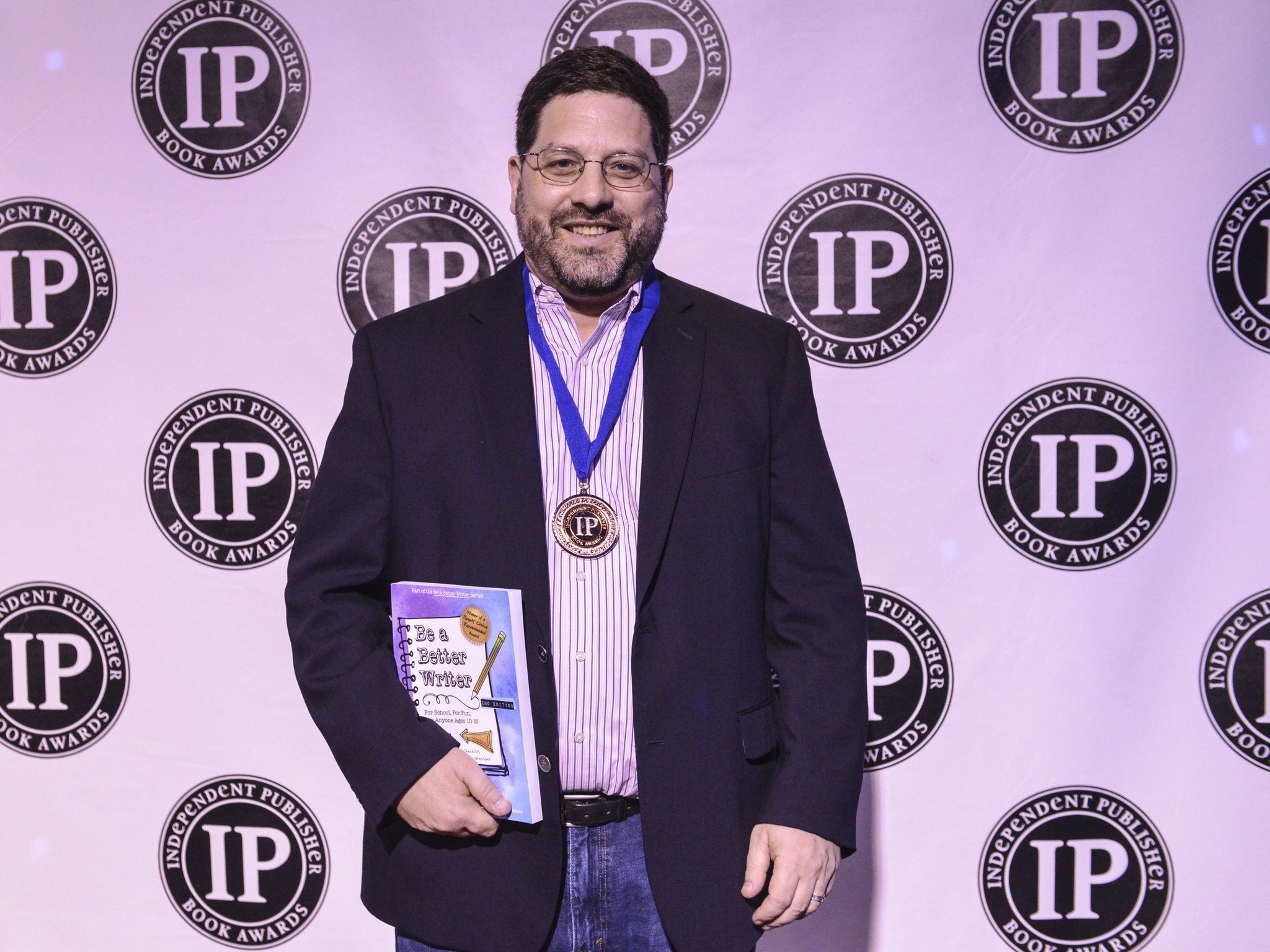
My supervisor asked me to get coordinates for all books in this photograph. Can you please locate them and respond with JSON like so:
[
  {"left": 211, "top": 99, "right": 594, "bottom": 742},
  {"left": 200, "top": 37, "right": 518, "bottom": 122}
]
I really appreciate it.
[{"left": 387, "top": 579, "right": 545, "bottom": 827}]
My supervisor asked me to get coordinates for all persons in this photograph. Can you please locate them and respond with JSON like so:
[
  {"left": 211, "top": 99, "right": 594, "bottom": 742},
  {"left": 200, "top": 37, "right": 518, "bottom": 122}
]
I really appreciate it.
[{"left": 285, "top": 45, "right": 867, "bottom": 952}]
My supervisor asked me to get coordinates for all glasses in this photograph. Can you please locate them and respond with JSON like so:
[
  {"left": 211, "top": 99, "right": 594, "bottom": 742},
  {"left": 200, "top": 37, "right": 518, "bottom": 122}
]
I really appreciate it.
[{"left": 521, "top": 146, "right": 665, "bottom": 189}]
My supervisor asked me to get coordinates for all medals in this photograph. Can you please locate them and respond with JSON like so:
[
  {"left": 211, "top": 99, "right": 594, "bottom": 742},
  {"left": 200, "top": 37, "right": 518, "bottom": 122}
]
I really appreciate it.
[{"left": 550, "top": 491, "right": 623, "bottom": 559}]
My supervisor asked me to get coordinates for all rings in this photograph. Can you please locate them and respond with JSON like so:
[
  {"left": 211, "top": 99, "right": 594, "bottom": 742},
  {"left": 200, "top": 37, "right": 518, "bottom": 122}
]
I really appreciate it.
[{"left": 812, "top": 892, "right": 825, "bottom": 903}]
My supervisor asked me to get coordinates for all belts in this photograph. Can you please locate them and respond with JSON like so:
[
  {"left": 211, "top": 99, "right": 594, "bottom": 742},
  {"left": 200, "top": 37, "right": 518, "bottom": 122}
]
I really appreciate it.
[{"left": 557, "top": 789, "right": 643, "bottom": 828}]
